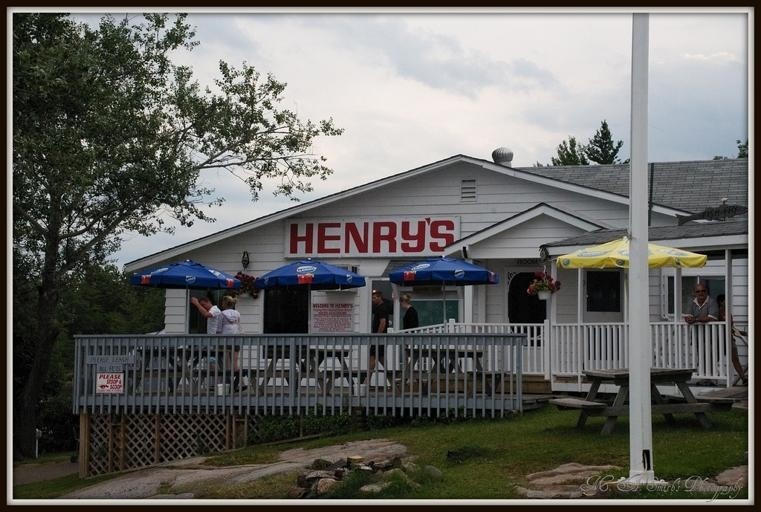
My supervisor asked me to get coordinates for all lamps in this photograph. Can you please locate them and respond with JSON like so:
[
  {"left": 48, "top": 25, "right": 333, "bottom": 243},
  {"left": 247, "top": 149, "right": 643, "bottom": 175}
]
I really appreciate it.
[{"left": 242, "top": 251, "right": 249, "bottom": 271}]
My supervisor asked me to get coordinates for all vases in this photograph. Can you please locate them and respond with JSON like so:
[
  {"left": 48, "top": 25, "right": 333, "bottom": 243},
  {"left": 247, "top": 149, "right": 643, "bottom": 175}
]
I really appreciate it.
[{"left": 538, "top": 290, "right": 551, "bottom": 300}]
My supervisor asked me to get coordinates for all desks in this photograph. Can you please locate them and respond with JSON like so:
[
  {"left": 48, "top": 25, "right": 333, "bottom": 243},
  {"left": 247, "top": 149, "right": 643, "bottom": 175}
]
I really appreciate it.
[{"left": 577, "top": 368, "right": 715, "bottom": 437}]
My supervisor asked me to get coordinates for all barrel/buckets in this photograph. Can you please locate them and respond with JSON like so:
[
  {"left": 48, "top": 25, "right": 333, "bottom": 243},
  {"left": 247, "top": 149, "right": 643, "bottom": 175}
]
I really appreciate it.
[
  {"left": 354, "top": 384, "right": 366, "bottom": 396},
  {"left": 217, "top": 383, "right": 231, "bottom": 396}
]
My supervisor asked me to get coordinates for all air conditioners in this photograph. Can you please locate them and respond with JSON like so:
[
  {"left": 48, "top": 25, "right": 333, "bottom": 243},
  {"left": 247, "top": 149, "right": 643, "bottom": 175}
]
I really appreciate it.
[{"left": 317, "top": 264, "right": 358, "bottom": 293}]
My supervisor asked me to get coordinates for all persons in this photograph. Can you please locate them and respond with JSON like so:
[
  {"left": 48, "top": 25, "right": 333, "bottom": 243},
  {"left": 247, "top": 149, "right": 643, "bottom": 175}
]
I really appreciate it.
[
  {"left": 716, "top": 294, "right": 748, "bottom": 385},
  {"left": 395, "top": 293, "right": 418, "bottom": 384},
  {"left": 363, "top": 290, "right": 390, "bottom": 385},
  {"left": 191, "top": 295, "right": 221, "bottom": 392},
  {"left": 217, "top": 296, "right": 247, "bottom": 393},
  {"left": 685, "top": 282, "right": 719, "bottom": 385}
]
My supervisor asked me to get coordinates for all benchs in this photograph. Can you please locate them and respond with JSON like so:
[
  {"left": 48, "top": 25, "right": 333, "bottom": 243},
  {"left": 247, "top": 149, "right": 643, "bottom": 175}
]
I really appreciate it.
[{"left": 549, "top": 398, "right": 741, "bottom": 437}]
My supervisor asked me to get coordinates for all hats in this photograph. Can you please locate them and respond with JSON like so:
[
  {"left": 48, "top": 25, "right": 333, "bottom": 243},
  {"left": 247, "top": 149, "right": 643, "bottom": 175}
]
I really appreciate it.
[{"left": 372, "top": 288, "right": 383, "bottom": 294}]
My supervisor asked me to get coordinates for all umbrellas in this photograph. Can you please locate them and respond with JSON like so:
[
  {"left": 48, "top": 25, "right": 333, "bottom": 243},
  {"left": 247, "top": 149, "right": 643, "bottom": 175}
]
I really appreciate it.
[
  {"left": 388, "top": 253, "right": 499, "bottom": 333},
  {"left": 257, "top": 257, "right": 366, "bottom": 334},
  {"left": 131, "top": 259, "right": 243, "bottom": 333},
  {"left": 556, "top": 235, "right": 708, "bottom": 322}
]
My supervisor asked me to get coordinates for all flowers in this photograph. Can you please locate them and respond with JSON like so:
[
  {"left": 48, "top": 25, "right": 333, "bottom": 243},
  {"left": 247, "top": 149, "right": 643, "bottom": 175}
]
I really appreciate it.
[
  {"left": 527, "top": 271, "right": 561, "bottom": 296},
  {"left": 230, "top": 271, "right": 255, "bottom": 295}
]
